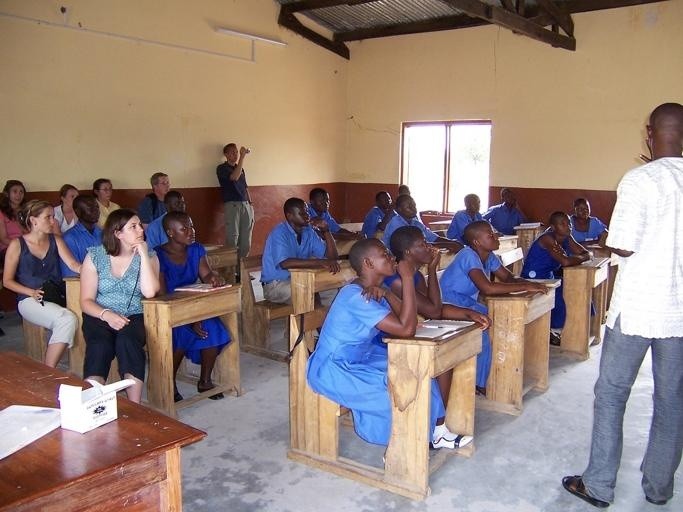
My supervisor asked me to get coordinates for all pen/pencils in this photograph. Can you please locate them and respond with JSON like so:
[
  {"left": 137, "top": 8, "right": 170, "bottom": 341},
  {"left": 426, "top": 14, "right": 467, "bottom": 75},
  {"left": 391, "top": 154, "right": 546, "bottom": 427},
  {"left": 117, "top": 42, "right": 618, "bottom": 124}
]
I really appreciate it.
[{"left": 425, "top": 325, "right": 444, "bottom": 329}]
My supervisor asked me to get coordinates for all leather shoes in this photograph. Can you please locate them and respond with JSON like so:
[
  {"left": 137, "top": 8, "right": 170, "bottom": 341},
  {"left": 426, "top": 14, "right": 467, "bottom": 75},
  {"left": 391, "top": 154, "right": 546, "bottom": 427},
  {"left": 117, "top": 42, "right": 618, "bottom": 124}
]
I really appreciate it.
[
  {"left": 432, "top": 434, "right": 474, "bottom": 450},
  {"left": 563, "top": 475, "right": 609, "bottom": 508}
]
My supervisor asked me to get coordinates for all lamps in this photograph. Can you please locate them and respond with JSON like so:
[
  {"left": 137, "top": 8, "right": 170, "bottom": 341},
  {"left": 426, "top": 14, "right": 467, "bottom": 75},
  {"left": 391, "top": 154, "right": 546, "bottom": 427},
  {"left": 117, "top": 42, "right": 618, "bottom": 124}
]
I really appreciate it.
[{"left": 215, "top": 25, "right": 288, "bottom": 48}]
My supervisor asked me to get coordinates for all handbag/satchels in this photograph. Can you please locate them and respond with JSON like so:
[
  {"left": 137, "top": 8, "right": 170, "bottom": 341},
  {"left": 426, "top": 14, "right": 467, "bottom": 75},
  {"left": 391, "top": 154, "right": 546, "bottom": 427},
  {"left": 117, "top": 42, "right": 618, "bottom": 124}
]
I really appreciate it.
[{"left": 41, "top": 280, "right": 66, "bottom": 307}]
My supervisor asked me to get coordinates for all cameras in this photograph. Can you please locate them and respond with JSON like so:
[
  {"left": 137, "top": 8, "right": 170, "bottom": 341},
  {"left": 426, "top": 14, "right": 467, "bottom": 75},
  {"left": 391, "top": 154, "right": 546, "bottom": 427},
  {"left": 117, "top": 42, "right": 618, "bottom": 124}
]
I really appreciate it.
[{"left": 241, "top": 146, "right": 251, "bottom": 153}]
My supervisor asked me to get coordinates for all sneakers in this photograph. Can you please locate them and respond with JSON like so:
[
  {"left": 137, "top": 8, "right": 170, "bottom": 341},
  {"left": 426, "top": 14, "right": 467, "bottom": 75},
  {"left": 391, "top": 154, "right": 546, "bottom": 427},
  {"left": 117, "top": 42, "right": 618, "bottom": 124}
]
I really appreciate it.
[
  {"left": 175, "top": 393, "right": 183, "bottom": 402},
  {"left": 198, "top": 387, "right": 223, "bottom": 399},
  {"left": 550, "top": 332, "right": 560, "bottom": 345}
]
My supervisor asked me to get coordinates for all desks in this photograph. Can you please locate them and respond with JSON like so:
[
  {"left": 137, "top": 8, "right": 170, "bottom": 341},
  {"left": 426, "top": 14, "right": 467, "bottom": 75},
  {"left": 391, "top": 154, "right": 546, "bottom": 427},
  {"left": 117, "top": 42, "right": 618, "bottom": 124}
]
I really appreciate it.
[{"left": 0, "top": 345, "right": 209, "bottom": 511}]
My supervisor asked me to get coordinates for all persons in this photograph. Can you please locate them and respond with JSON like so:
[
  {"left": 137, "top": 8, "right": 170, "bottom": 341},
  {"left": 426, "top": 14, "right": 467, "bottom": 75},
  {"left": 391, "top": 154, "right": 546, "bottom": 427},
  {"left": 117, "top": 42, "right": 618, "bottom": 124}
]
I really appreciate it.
[
  {"left": 519, "top": 195, "right": 606, "bottom": 345},
  {"left": 307, "top": 186, "right": 525, "bottom": 255},
  {"left": 561, "top": 102, "right": 683, "bottom": 507},
  {"left": 216, "top": 143, "right": 255, "bottom": 284},
  {"left": 307, "top": 237, "right": 473, "bottom": 465},
  {"left": 260, "top": 198, "right": 340, "bottom": 354},
  {"left": 437, "top": 220, "right": 549, "bottom": 391},
  {"left": 0, "top": 173, "right": 234, "bottom": 402},
  {"left": 383, "top": 225, "right": 492, "bottom": 411}
]
